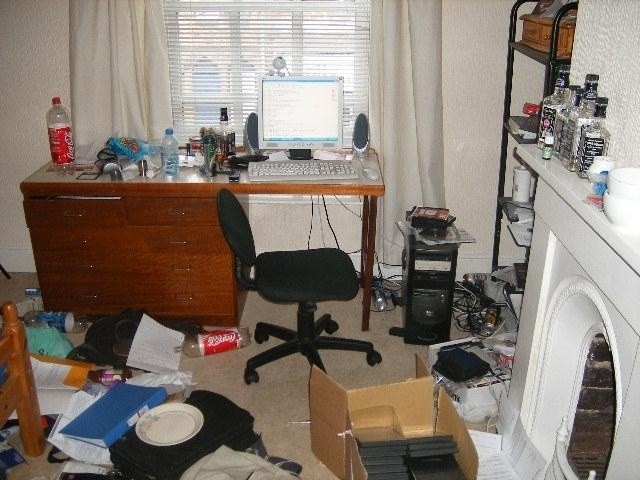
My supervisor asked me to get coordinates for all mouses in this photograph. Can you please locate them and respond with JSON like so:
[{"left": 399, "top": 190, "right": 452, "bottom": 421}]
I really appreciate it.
[{"left": 361, "top": 168, "right": 379, "bottom": 181}]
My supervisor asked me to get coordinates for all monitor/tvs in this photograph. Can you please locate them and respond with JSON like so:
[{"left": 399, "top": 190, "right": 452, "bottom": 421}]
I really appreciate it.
[{"left": 258, "top": 75, "right": 344, "bottom": 160}]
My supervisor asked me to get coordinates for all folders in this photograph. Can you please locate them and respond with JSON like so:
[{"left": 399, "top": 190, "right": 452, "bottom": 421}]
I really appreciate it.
[{"left": 59, "top": 381, "right": 168, "bottom": 449}]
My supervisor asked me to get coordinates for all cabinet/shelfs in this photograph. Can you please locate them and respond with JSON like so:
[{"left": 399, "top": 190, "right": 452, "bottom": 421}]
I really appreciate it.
[{"left": 492, "top": 0, "right": 579, "bottom": 285}]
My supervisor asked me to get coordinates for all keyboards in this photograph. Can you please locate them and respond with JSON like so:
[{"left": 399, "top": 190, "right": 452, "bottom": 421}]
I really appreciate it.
[{"left": 248, "top": 160, "right": 359, "bottom": 180}]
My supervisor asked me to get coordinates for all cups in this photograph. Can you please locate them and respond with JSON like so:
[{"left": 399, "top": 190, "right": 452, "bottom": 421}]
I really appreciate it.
[{"left": 512, "top": 164, "right": 532, "bottom": 204}]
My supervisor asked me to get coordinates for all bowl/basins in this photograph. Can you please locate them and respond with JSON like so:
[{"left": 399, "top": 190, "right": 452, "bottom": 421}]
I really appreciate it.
[
  {"left": 602, "top": 189, "right": 640, "bottom": 229},
  {"left": 606, "top": 167, "right": 640, "bottom": 198}
]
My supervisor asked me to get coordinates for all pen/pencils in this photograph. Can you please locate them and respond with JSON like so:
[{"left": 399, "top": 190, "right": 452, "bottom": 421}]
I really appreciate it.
[{"left": 287, "top": 420, "right": 310, "bottom": 423}]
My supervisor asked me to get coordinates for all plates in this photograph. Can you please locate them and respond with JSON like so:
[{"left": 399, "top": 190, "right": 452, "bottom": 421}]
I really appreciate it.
[{"left": 135, "top": 402, "right": 205, "bottom": 448}]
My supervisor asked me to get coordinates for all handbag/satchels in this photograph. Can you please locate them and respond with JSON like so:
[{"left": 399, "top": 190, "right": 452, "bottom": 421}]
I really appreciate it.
[
  {"left": 109, "top": 390, "right": 257, "bottom": 479},
  {"left": 80, "top": 318, "right": 124, "bottom": 368}
]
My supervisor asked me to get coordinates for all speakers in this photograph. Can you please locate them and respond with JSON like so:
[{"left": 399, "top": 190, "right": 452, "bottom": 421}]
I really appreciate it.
[
  {"left": 352, "top": 113, "right": 370, "bottom": 160},
  {"left": 243, "top": 112, "right": 260, "bottom": 156}
]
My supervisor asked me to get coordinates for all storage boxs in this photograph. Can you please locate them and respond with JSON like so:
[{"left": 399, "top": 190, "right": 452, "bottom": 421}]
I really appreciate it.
[
  {"left": 519, "top": 13, "right": 576, "bottom": 59},
  {"left": 309, "top": 352, "right": 479, "bottom": 480}
]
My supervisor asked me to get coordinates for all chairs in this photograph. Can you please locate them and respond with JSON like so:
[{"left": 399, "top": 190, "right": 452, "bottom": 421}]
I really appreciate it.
[{"left": 217, "top": 188, "right": 383, "bottom": 385}]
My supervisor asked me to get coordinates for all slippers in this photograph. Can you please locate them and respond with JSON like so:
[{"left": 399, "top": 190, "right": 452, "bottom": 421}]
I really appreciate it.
[{"left": 113, "top": 310, "right": 144, "bottom": 357}]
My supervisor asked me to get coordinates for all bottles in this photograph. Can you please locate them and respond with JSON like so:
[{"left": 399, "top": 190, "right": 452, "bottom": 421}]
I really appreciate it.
[
  {"left": 17, "top": 309, "right": 88, "bottom": 335},
  {"left": 538, "top": 64, "right": 612, "bottom": 181},
  {"left": 173, "top": 325, "right": 253, "bottom": 358},
  {"left": 202, "top": 134, "right": 216, "bottom": 178},
  {"left": 88, "top": 369, "right": 133, "bottom": 385},
  {"left": 219, "top": 107, "right": 230, "bottom": 158},
  {"left": 44, "top": 96, "right": 76, "bottom": 176},
  {"left": 160, "top": 128, "right": 181, "bottom": 176}
]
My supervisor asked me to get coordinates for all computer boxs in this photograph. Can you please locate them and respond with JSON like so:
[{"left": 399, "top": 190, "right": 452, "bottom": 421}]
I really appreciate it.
[{"left": 402, "top": 210, "right": 458, "bottom": 345}]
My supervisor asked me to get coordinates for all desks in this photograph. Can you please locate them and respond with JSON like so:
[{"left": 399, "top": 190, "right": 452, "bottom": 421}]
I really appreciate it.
[{"left": 20, "top": 145, "right": 385, "bottom": 331}]
